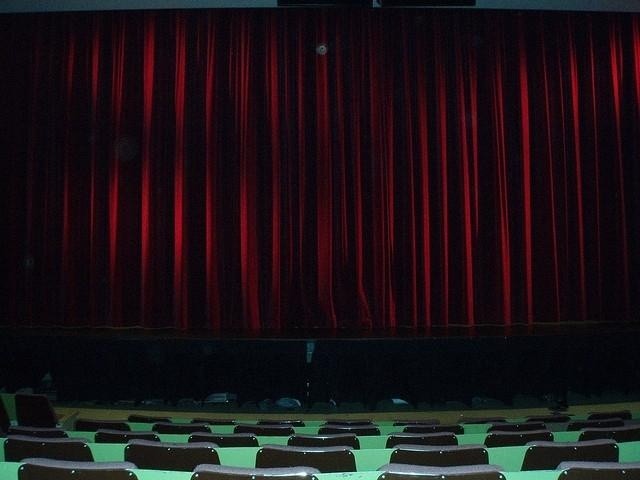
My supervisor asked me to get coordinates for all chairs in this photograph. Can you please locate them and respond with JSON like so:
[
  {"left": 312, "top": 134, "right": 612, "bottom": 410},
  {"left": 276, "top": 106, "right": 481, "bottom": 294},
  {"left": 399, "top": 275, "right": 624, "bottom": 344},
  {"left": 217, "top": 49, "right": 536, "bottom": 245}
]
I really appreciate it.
[{"left": 0, "top": 396, "right": 639, "bottom": 479}]
